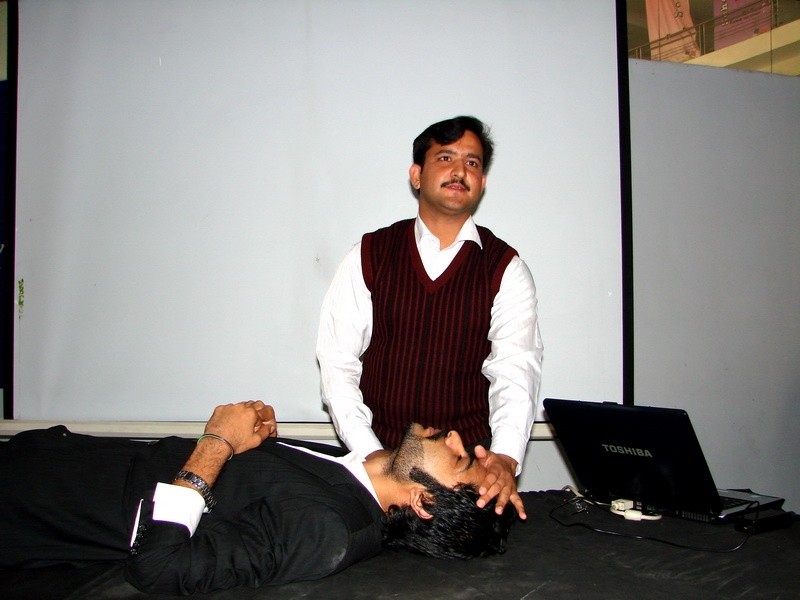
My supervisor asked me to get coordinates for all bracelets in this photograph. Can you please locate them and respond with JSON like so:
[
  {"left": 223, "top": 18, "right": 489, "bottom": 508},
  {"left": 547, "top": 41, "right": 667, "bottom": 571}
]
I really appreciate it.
[
  {"left": 198, "top": 434, "right": 235, "bottom": 460},
  {"left": 175, "top": 472, "right": 216, "bottom": 514}
]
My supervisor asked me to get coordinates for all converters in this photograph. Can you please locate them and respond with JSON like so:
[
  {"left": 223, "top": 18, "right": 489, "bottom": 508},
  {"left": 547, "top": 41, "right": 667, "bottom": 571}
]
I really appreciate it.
[{"left": 735, "top": 510, "right": 792, "bottom": 534}]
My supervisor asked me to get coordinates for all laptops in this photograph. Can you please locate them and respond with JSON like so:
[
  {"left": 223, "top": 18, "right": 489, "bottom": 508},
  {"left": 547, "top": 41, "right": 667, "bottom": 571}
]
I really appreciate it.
[{"left": 543, "top": 398, "right": 785, "bottom": 524}]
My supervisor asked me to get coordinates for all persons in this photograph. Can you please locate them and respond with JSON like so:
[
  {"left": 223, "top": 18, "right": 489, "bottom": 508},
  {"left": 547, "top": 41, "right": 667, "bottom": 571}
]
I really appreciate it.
[
  {"left": 0, "top": 401, "right": 510, "bottom": 600},
  {"left": 314, "top": 114, "right": 544, "bottom": 520}
]
